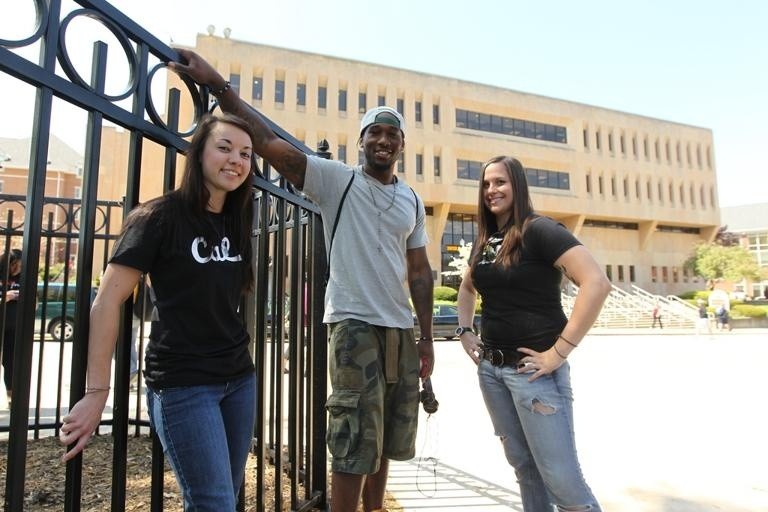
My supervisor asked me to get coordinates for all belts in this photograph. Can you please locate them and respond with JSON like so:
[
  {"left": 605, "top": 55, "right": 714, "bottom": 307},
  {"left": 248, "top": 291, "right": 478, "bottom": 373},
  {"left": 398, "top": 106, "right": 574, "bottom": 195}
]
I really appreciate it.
[{"left": 481, "top": 347, "right": 523, "bottom": 367}]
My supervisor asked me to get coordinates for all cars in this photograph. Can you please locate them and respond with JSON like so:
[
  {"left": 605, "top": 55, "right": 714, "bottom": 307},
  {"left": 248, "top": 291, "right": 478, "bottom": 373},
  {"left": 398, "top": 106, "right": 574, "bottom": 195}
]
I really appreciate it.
[{"left": 412, "top": 305, "right": 482, "bottom": 339}]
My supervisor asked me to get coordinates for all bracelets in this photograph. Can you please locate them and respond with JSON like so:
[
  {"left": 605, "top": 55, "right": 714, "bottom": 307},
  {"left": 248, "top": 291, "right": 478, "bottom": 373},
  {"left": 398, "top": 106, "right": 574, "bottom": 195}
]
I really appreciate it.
[
  {"left": 558, "top": 334, "right": 576, "bottom": 348},
  {"left": 553, "top": 344, "right": 567, "bottom": 360},
  {"left": 86, "top": 387, "right": 109, "bottom": 395},
  {"left": 209, "top": 81, "right": 231, "bottom": 103}
]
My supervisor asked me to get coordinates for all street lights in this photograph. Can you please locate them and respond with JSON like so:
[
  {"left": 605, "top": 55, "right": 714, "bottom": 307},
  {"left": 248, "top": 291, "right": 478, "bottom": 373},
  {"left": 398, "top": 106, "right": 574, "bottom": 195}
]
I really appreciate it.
[
  {"left": 47, "top": 161, "right": 60, "bottom": 266},
  {"left": 1, "top": 158, "right": 11, "bottom": 162}
]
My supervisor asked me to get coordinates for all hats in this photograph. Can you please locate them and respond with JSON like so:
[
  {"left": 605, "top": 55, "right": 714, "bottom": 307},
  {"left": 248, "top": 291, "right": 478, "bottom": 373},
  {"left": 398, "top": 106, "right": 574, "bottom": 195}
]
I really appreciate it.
[{"left": 357, "top": 107, "right": 405, "bottom": 152}]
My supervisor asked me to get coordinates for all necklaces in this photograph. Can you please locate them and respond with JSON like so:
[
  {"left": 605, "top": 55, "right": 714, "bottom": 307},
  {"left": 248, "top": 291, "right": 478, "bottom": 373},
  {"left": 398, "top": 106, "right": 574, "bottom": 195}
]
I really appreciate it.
[
  {"left": 360, "top": 165, "right": 396, "bottom": 252},
  {"left": 205, "top": 211, "right": 229, "bottom": 258},
  {"left": 483, "top": 223, "right": 506, "bottom": 253}
]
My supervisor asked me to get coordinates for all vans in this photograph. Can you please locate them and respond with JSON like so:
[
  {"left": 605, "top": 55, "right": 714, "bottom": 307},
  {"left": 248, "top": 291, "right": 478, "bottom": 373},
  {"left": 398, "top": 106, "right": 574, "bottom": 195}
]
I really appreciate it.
[{"left": 32, "top": 283, "right": 98, "bottom": 341}]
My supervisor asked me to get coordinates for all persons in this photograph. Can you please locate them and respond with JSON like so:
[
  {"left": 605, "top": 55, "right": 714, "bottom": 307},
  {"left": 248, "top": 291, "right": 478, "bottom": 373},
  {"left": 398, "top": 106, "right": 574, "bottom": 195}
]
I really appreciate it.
[
  {"left": 1, "top": 249, "right": 39, "bottom": 410},
  {"left": 456, "top": 155, "right": 612, "bottom": 512},
  {"left": 652, "top": 303, "right": 663, "bottom": 329},
  {"left": 697, "top": 298, "right": 712, "bottom": 334},
  {"left": 59, "top": 112, "right": 258, "bottom": 512},
  {"left": 50, "top": 254, "right": 75, "bottom": 282},
  {"left": 719, "top": 304, "right": 732, "bottom": 331},
  {"left": 158, "top": 46, "right": 435, "bottom": 511}
]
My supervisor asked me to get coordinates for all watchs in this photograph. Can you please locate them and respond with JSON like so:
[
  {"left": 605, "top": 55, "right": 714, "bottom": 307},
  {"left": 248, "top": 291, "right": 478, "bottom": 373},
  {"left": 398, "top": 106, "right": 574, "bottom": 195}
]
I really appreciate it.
[
  {"left": 455, "top": 326, "right": 475, "bottom": 338},
  {"left": 420, "top": 336, "right": 432, "bottom": 341}
]
